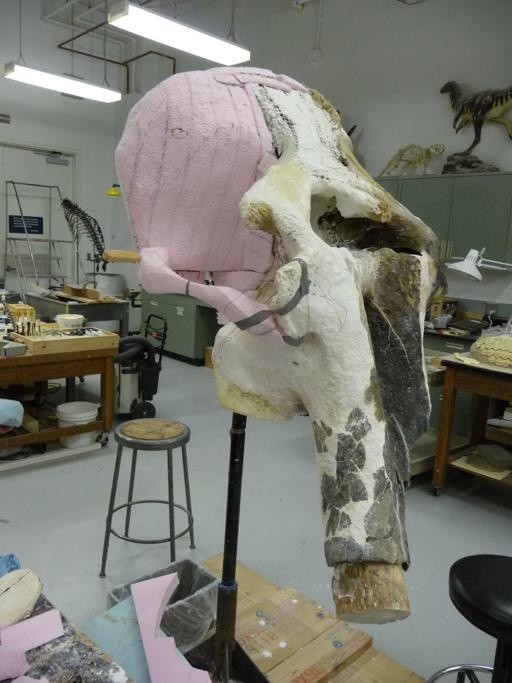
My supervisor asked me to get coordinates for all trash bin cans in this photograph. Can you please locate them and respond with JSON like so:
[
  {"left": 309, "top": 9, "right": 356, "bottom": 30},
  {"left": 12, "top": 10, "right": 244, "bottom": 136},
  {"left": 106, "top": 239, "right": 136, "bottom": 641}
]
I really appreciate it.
[{"left": 107, "top": 559, "right": 221, "bottom": 672}]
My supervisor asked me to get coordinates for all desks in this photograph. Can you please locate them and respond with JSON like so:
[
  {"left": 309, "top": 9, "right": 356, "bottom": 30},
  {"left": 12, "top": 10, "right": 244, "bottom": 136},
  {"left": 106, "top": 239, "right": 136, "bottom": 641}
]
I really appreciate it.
[
  {"left": 0, "top": 321, "right": 119, "bottom": 452},
  {"left": 430, "top": 350, "right": 512, "bottom": 497}
]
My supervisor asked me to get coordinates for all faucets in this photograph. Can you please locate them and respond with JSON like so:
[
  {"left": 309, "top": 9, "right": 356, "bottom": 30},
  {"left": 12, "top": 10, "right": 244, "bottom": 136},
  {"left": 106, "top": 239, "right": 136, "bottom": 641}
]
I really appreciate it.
[{"left": 82, "top": 280, "right": 97, "bottom": 294}]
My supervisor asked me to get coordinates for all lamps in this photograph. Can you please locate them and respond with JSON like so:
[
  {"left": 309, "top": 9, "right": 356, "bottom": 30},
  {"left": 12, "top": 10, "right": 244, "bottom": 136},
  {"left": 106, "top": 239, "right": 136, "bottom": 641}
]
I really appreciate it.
[
  {"left": 2, "top": 0, "right": 251, "bottom": 104},
  {"left": 446, "top": 247, "right": 511, "bottom": 283}
]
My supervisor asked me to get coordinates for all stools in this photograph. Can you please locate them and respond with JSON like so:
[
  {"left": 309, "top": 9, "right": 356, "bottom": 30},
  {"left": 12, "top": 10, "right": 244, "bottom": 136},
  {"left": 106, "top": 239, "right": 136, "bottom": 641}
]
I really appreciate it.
[
  {"left": 99, "top": 417, "right": 195, "bottom": 578},
  {"left": 426, "top": 554, "right": 512, "bottom": 682}
]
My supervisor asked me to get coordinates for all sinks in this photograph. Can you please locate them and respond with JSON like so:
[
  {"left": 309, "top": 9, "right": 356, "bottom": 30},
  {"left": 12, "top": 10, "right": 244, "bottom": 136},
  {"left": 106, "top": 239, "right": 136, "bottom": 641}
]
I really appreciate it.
[{"left": 26, "top": 292, "right": 87, "bottom": 321}]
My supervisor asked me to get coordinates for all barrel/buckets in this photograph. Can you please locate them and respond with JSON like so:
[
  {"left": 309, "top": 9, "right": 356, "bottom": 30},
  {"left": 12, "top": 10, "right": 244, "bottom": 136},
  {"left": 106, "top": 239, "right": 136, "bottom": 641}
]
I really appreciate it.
[{"left": 56, "top": 402, "right": 99, "bottom": 448}]
